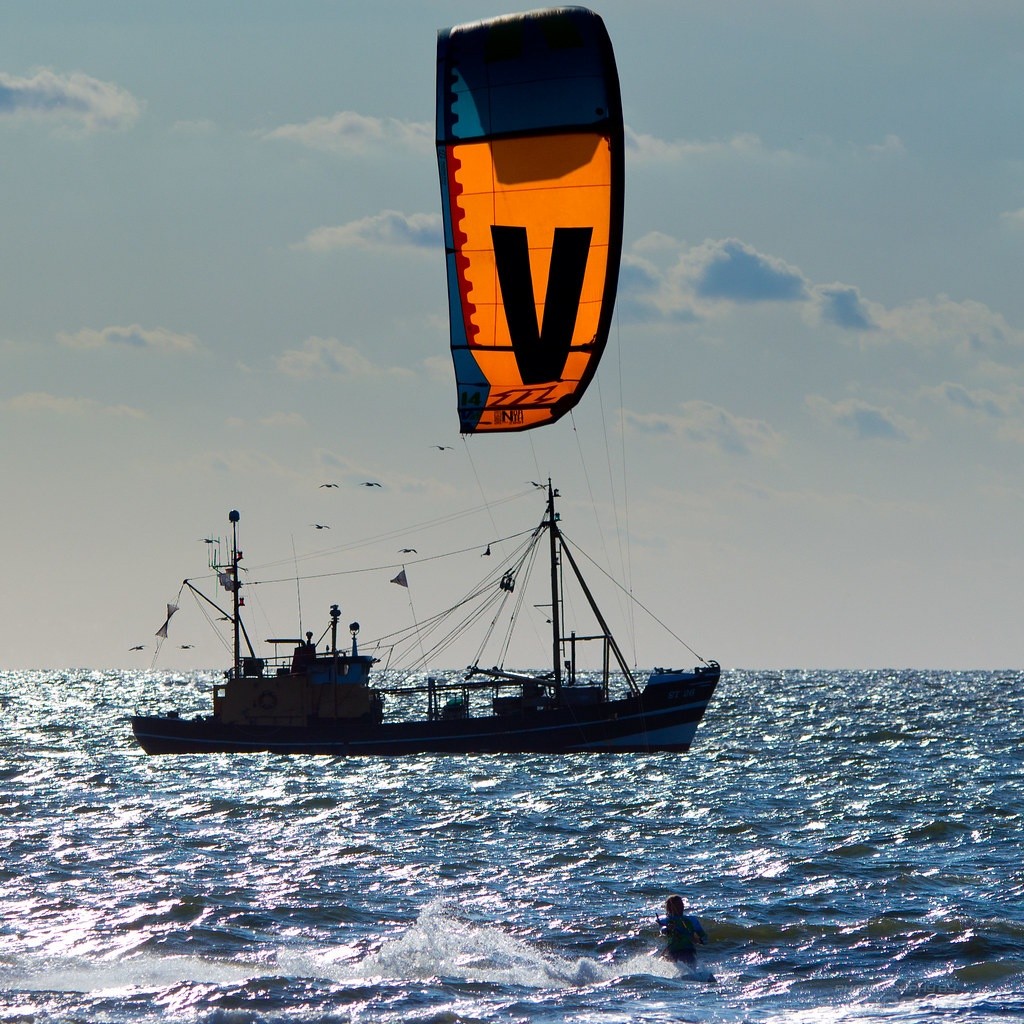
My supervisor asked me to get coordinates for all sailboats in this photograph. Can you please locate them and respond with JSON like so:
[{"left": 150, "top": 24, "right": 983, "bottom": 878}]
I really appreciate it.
[{"left": 131, "top": 475, "right": 723, "bottom": 753}]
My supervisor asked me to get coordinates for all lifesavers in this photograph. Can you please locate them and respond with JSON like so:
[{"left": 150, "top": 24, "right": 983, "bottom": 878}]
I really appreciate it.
[{"left": 258, "top": 692, "right": 277, "bottom": 710}]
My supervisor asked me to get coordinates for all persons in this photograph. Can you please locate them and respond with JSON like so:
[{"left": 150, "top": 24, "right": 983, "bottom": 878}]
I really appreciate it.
[{"left": 657, "top": 895, "right": 710, "bottom": 970}]
